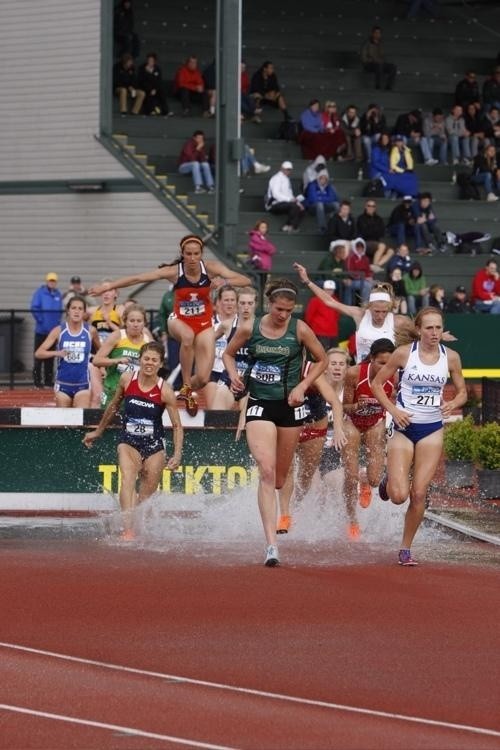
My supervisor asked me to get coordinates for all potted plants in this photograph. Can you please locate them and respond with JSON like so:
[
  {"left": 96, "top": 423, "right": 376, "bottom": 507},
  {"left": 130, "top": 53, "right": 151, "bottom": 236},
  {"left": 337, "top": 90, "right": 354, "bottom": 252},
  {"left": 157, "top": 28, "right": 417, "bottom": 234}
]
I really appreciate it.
[
  {"left": 436, "top": 419, "right": 479, "bottom": 494},
  {"left": 471, "top": 418, "right": 500, "bottom": 503}
]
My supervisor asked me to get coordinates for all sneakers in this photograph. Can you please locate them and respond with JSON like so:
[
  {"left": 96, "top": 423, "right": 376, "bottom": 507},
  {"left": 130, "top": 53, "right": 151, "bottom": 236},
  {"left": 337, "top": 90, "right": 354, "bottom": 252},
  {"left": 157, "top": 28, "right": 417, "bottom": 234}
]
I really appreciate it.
[
  {"left": 348, "top": 524, "right": 360, "bottom": 539},
  {"left": 176, "top": 389, "right": 197, "bottom": 416},
  {"left": 398, "top": 550, "right": 419, "bottom": 566},
  {"left": 264, "top": 544, "right": 279, "bottom": 565},
  {"left": 379, "top": 474, "right": 389, "bottom": 501},
  {"left": 276, "top": 516, "right": 291, "bottom": 535},
  {"left": 359, "top": 482, "right": 371, "bottom": 508}
]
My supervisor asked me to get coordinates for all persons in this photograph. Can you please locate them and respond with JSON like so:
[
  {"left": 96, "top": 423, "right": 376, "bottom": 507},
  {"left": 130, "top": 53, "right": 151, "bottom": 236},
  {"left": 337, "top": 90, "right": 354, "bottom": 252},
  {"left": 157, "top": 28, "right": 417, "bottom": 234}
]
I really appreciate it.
[
  {"left": 370, "top": 307, "right": 468, "bottom": 567},
  {"left": 294, "top": 69, "right": 499, "bottom": 320},
  {"left": 28, "top": 221, "right": 276, "bottom": 416},
  {"left": 221, "top": 281, "right": 329, "bottom": 567},
  {"left": 358, "top": 28, "right": 397, "bottom": 90},
  {"left": 178, "top": 130, "right": 220, "bottom": 194},
  {"left": 209, "top": 144, "right": 271, "bottom": 179},
  {"left": 112, "top": 35, "right": 297, "bottom": 126},
  {"left": 276, "top": 261, "right": 457, "bottom": 546},
  {"left": 264, "top": 161, "right": 305, "bottom": 233},
  {"left": 82, "top": 343, "right": 184, "bottom": 544}
]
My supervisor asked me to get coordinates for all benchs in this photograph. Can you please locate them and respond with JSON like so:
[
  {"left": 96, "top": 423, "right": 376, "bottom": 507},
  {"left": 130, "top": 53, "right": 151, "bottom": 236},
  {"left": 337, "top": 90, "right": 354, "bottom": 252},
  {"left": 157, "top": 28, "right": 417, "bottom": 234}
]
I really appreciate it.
[{"left": 111, "top": 1, "right": 500, "bottom": 311}]
